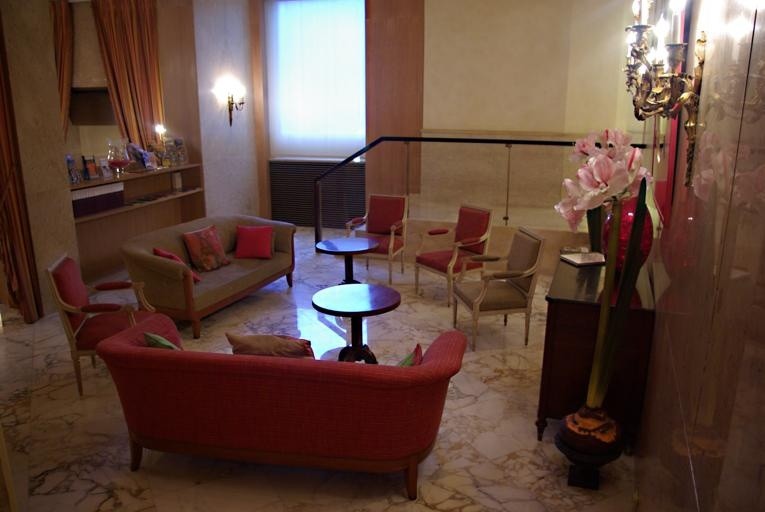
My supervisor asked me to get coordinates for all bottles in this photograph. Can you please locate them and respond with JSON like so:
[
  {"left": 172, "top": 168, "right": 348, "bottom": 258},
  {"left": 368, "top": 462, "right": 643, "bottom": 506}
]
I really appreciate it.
[
  {"left": 106, "top": 143, "right": 130, "bottom": 179},
  {"left": 160, "top": 144, "right": 189, "bottom": 170}
]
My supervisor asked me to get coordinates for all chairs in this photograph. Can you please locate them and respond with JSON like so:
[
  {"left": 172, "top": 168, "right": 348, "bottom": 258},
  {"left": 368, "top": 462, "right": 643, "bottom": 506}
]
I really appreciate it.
[
  {"left": 415, "top": 203, "right": 494, "bottom": 307},
  {"left": 42, "top": 249, "right": 157, "bottom": 397},
  {"left": 345, "top": 193, "right": 409, "bottom": 285},
  {"left": 451, "top": 225, "right": 548, "bottom": 351}
]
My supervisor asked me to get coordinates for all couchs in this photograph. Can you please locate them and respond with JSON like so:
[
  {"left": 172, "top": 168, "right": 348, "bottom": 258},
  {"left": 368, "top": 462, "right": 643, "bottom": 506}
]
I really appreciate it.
[
  {"left": 95, "top": 312, "right": 468, "bottom": 501},
  {"left": 223, "top": 330, "right": 315, "bottom": 359},
  {"left": 119, "top": 213, "right": 296, "bottom": 339}
]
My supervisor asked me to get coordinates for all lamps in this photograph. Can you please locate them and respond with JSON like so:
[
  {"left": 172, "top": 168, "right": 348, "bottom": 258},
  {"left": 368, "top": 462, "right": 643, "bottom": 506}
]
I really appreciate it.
[{"left": 622, "top": 0, "right": 708, "bottom": 188}]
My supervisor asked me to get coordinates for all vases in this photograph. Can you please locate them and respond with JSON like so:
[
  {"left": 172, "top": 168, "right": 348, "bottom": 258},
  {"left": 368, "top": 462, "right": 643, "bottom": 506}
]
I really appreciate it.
[
  {"left": 600, "top": 196, "right": 654, "bottom": 274},
  {"left": 662, "top": 210, "right": 706, "bottom": 287}
]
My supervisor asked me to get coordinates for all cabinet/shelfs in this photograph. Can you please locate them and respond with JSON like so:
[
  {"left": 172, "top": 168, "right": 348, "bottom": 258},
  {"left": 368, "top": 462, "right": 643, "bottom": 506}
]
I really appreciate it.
[{"left": 69, "top": 160, "right": 206, "bottom": 285}]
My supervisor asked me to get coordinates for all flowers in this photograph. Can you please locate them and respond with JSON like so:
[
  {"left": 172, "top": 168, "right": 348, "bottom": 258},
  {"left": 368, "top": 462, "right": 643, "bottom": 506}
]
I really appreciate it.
[
  {"left": 657, "top": 130, "right": 765, "bottom": 490},
  {"left": 553, "top": 128, "right": 655, "bottom": 468}
]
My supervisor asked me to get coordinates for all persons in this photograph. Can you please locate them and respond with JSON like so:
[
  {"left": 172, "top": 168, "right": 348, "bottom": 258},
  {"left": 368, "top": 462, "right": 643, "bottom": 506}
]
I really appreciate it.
[{"left": 560, "top": 252, "right": 605, "bottom": 268}]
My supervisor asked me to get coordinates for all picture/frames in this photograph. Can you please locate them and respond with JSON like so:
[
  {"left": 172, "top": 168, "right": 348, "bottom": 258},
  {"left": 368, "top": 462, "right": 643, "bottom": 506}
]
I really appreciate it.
[
  {"left": 163, "top": 135, "right": 189, "bottom": 166},
  {"left": 81, "top": 155, "right": 99, "bottom": 180}
]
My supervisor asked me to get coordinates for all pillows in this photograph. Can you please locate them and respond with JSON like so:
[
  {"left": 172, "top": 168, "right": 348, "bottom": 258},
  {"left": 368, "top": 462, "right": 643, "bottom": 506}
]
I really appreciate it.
[
  {"left": 152, "top": 247, "right": 202, "bottom": 282},
  {"left": 180, "top": 224, "right": 231, "bottom": 272},
  {"left": 143, "top": 331, "right": 180, "bottom": 351},
  {"left": 377, "top": 342, "right": 422, "bottom": 368},
  {"left": 233, "top": 223, "right": 273, "bottom": 259}
]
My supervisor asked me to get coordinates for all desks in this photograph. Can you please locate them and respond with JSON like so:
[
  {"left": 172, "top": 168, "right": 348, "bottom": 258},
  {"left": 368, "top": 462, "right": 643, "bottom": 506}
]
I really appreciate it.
[
  {"left": 535, "top": 248, "right": 656, "bottom": 455},
  {"left": 316, "top": 236, "right": 379, "bottom": 285},
  {"left": 311, "top": 283, "right": 401, "bottom": 364}
]
